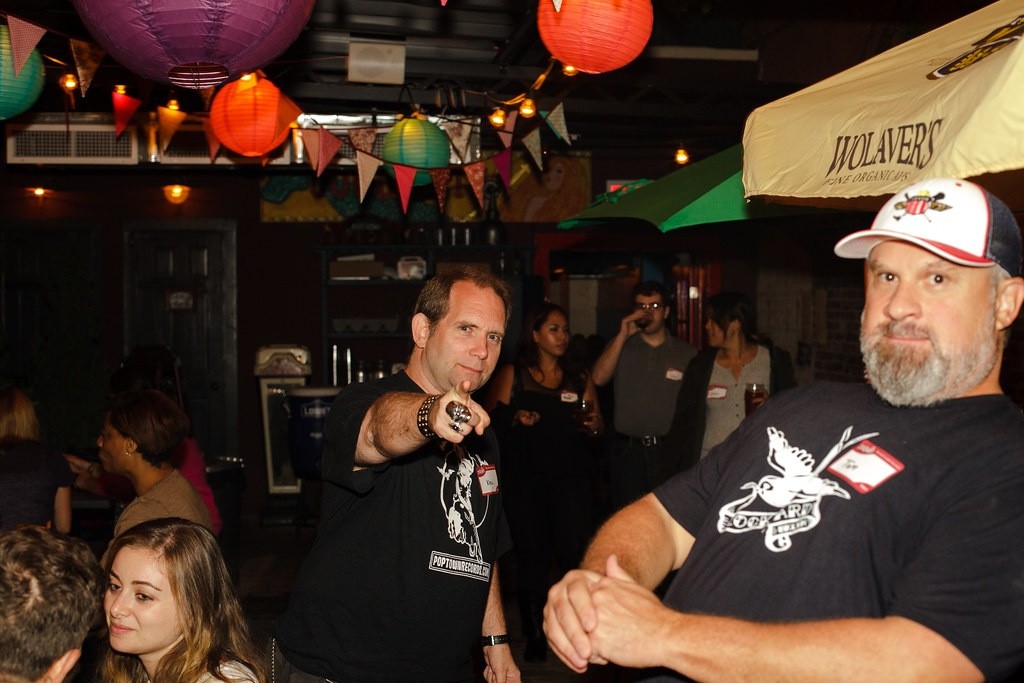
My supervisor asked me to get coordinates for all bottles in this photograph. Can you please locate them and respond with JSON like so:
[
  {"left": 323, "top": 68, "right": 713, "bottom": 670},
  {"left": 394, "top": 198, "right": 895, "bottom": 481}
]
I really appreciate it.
[
  {"left": 476, "top": 207, "right": 508, "bottom": 245},
  {"left": 354, "top": 360, "right": 367, "bottom": 384}
]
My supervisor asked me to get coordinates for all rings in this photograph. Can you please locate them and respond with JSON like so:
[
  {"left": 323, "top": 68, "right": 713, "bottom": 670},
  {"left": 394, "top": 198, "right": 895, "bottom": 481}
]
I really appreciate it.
[
  {"left": 594, "top": 430, "right": 598, "bottom": 434},
  {"left": 449, "top": 422, "right": 462, "bottom": 432},
  {"left": 446, "top": 401, "right": 471, "bottom": 423}
]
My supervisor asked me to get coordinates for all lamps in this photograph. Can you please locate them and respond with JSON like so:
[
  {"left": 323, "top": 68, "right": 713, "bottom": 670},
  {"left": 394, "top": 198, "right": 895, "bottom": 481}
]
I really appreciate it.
[{"left": 164, "top": 184, "right": 189, "bottom": 204}]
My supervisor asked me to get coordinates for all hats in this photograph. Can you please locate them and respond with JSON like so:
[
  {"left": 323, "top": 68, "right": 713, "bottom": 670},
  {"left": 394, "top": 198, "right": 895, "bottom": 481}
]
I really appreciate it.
[{"left": 834, "top": 177, "right": 1022, "bottom": 277}]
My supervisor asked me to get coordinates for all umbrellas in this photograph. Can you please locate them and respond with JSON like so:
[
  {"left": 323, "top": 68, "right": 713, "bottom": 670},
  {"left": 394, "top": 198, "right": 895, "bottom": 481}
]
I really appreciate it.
[
  {"left": 559, "top": 142, "right": 792, "bottom": 233},
  {"left": 744, "top": 0, "right": 1024, "bottom": 214}
]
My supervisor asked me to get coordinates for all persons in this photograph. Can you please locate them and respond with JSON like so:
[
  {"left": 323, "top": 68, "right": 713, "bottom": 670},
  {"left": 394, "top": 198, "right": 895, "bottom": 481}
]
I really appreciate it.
[
  {"left": 544, "top": 177, "right": 1024, "bottom": 683},
  {"left": 590, "top": 280, "right": 699, "bottom": 516},
  {"left": 0, "top": 379, "right": 271, "bottom": 683},
  {"left": 666, "top": 292, "right": 797, "bottom": 476},
  {"left": 484, "top": 302, "right": 604, "bottom": 590},
  {"left": 261, "top": 262, "right": 521, "bottom": 683}
]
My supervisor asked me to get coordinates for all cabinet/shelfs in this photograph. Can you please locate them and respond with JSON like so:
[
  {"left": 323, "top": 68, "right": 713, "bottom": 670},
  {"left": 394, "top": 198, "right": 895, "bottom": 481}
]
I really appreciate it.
[{"left": 323, "top": 245, "right": 526, "bottom": 391}]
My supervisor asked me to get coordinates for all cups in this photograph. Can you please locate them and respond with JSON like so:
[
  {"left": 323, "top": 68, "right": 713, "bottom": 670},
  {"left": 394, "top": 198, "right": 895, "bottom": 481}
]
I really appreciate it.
[
  {"left": 575, "top": 399, "right": 593, "bottom": 432},
  {"left": 634, "top": 312, "right": 653, "bottom": 329},
  {"left": 744, "top": 383, "right": 764, "bottom": 416},
  {"left": 434, "top": 227, "right": 475, "bottom": 245}
]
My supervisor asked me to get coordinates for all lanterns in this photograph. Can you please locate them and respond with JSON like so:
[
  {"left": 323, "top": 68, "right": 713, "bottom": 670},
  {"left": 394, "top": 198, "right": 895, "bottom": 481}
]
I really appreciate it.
[
  {"left": 383, "top": 116, "right": 451, "bottom": 185},
  {"left": 74, "top": 0, "right": 313, "bottom": 91},
  {"left": 537, "top": 0, "right": 653, "bottom": 75},
  {"left": 0, "top": 25, "right": 46, "bottom": 121},
  {"left": 208, "top": 75, "right": 290, "bottom": 157}
]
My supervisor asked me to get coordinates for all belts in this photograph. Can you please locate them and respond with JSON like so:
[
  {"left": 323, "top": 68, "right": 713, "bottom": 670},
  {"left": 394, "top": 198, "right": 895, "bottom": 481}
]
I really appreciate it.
[{"left": 616, "top": 431, "right": 666, "bottom": 448}]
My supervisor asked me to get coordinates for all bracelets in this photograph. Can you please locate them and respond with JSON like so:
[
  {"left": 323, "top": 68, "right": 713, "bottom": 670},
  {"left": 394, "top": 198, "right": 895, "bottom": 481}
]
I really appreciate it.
[
  {"left": 481, "top": 635, "right": 513, "bottom": 646},
  {"left": 416, "top": 394, "right": 441, "bottom": 440}
]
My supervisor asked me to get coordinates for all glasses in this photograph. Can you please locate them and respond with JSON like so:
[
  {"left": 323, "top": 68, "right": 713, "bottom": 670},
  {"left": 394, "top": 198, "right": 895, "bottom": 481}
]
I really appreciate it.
[{"left": 635, "top": 302, "right": 665, "bottom": 309}]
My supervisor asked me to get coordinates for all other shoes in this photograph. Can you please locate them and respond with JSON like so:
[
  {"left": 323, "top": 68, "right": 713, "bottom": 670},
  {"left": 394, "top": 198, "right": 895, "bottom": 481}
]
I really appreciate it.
[{"left": 525, "top": 638, "right": 548, "bottom": 662}]
